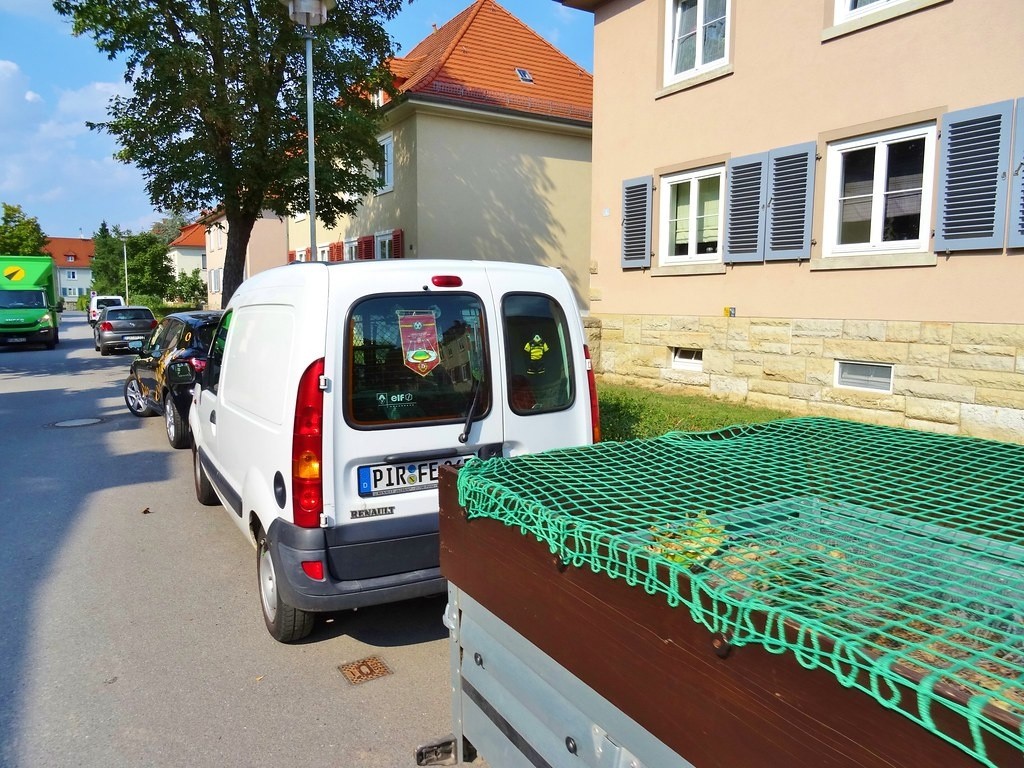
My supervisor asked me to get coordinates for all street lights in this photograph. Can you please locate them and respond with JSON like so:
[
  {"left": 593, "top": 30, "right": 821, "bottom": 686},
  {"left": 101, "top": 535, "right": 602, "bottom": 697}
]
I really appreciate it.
[
  {"left": 277, "top": 0, "right": 336, "bottom": 263},
  {"left": 119, "top": 234, "right": 129, "bottom": 306}
]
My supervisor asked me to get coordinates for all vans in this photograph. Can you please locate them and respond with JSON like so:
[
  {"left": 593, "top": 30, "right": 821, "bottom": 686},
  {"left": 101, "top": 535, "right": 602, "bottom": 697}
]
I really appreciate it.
[{"left": 164, "top": 257, "right": 601, "bottom": 643}]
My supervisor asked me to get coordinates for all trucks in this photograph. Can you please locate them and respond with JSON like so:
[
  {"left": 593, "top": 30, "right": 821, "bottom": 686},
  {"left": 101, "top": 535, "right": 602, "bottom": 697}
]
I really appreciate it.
[{"left": 0, "top": 256, "right": 66, "bottom": 349}]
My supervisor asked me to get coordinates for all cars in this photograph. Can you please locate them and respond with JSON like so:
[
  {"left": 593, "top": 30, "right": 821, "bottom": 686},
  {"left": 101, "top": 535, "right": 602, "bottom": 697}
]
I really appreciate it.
[
  {"left": 122, "top": 310, "right": 232, "bottom": 450},
  {"left": 93, "top": 306, "right": 159, "bottom": 356},
  {"left": 88, "top": 295, "right": 126, "bottom": 330}
]
